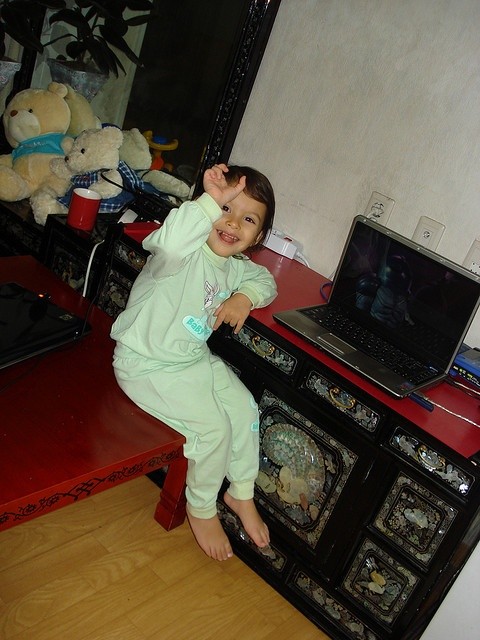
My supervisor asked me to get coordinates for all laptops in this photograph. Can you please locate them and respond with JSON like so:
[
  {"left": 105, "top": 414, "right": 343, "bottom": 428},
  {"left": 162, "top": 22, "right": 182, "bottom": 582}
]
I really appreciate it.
[{"left": 275, "top": 213, "right": 480, "bottom": 399}]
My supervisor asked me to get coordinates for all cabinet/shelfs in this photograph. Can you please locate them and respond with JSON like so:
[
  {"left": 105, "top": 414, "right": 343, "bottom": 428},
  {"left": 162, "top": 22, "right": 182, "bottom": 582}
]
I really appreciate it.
[
  {"left": 87, "top": 217, "right": 480, "bottom": 639},
  {"left": 0, "top": 201, "right": 106, "bottom": 307}
]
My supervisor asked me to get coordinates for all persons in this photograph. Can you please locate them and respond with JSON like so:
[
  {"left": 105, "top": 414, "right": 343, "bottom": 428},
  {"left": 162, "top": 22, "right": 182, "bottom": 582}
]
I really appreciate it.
[{"left": 110, "top": 163, "right": 278, "bottom": 561}]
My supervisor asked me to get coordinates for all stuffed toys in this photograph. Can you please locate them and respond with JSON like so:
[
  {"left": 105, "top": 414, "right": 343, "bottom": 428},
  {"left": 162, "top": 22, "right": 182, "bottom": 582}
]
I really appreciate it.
[
  {"left": 119, "top": 129, "right": 190, "bottom": 201},
  {"left": 50, "top": 125, "right": 123, "bottom": 211},
  {"left": 64, "top": 82, "right": 101, "bottom": 138},
  {"left": 142, "top": 129, "right": 178, "bottom": 173},
  {"left": 0, "top": 82, "right": 70, "bottom": 226}
]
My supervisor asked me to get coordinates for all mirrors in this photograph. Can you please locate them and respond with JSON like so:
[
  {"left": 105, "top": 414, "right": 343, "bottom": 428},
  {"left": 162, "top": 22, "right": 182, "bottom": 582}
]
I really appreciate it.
[{"left": 10, "top": 0, "right": 283, "bottom": 232}]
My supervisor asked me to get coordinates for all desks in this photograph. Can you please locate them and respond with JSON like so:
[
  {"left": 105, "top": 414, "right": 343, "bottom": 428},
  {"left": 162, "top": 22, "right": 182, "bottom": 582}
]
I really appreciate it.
[{"left": 0, "top": 251, "right": 185, "bottom": 535}]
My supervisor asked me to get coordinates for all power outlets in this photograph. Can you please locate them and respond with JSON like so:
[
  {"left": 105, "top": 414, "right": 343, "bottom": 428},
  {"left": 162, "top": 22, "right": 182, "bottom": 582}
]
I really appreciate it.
[
  {"left": 363, "top": 190, "right": 397, "bottom": 229},
  {"left": 460, "top": 240, "right": 479, "bottom": 278}
]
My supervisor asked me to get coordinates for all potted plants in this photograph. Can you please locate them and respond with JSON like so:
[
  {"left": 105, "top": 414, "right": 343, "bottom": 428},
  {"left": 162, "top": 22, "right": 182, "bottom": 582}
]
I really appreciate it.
[
  {"left": 1, "top": 0, "right": 45, "bottom": 99},
  {"left": 44, "top": 0, "right": 156, "bottom": 105}
]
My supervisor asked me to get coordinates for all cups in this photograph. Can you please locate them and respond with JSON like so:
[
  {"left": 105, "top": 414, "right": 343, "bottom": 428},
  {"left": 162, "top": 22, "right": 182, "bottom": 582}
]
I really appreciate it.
[{"left": 67, "top": 188, "right": 102, "bottom": 231}]
